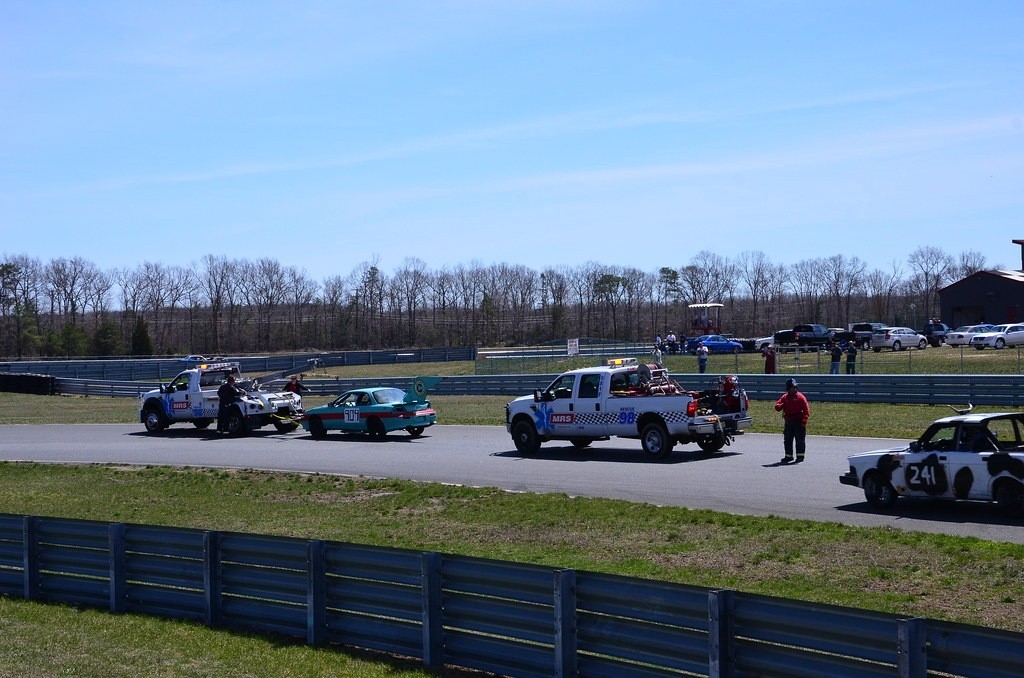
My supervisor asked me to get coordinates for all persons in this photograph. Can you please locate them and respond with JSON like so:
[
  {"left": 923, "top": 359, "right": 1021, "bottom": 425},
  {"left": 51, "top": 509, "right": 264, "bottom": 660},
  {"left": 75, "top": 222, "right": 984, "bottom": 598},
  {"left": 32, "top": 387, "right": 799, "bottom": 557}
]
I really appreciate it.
[
  {"left": 845, "top": 340, "right": 857, "bottom": 373},
  {"left": 278, "top": 376, "right": 311, "bottom": 399},
  {"left": 680, "top": 330, "right": 686, "bottom": 355},
  {"left": 830, "top": 340, "right": 843, "bottom": 374},
  {"left": 656, "top": 332, "right": 663, "bottom": 349},
  {"left": 775, "top": 379, "right": 810, "bottom": 463},
  {"left": 762, "top": 344, "right": 777, "bottom": 374},
  {"left": 929, "top": 318, "right": 941, "bottom": 324},
  {"left": 216, "top": 377, "right": 245, "bottom": 434},
  {"left": 696, "top": 342, "right": 709, "bottom": 373},
  {"left": 652, "top": 345, "right": 662, "bottom": 365},
  {"left": 694, "top": 313, "right": 706, "bottom": 326},
  {"left": 667, "top": 330, "right": 677, "bottom": 355}
]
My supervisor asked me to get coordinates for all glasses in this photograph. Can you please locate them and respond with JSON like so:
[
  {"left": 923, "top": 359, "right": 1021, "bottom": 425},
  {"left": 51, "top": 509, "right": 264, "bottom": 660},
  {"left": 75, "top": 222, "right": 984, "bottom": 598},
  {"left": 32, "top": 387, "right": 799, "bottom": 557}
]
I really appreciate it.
[{"left": 290, "top": 378, "right": 296, "bottom": 380}]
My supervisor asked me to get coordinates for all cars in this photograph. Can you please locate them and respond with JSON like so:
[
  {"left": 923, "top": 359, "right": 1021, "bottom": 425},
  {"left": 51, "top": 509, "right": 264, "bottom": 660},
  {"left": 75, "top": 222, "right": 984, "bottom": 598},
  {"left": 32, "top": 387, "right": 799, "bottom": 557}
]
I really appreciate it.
[
  {"left": 685, "top": 334, "right": 744, "bottom": 354},
  {"left": 944, "top": 322, "right": 1024, "bottom": 350},
  {"left": 177, "top": 355, "right": 206, "bottom": 361},
  {"left": 205, "top": 356, "right": 228, "bottom": 362}
]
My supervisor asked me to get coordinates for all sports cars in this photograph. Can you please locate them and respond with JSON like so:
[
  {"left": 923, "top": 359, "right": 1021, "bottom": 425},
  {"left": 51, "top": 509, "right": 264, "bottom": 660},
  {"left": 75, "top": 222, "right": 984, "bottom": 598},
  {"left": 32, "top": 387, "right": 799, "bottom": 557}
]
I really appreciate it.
[{"left": 299, "top": 374, "right": 444, "bottom": 441}]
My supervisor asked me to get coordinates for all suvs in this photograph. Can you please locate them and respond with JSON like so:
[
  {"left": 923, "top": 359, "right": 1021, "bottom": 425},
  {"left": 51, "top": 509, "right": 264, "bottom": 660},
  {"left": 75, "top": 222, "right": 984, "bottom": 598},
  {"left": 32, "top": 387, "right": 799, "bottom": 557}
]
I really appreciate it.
[{"left": 870, "top": 326, "right": 929, "bottom": 352}]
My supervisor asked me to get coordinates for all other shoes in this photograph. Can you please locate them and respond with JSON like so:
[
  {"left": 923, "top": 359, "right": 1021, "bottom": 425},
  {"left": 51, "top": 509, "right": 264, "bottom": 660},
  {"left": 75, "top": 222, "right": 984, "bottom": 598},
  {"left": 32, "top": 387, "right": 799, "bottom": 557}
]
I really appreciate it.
[
  {"left": 781, "top": 456, "right": 794, "bottom": 463},
  {"left": 795, "top": 455, "right": 804, "bottom": 462}
]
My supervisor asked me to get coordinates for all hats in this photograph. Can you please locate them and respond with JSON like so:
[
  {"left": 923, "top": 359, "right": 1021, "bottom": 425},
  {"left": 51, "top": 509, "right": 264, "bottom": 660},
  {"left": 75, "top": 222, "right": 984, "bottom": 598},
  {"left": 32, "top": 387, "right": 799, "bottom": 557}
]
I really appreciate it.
[
  {"left": 697, "top": 342, "right": 703, "bottom": 346},
  {"left": 785, "top": 378, "right": 798, "bottom": 388},
  {"left": 768, "top": 344, "right": 773, "bottom": 348}
]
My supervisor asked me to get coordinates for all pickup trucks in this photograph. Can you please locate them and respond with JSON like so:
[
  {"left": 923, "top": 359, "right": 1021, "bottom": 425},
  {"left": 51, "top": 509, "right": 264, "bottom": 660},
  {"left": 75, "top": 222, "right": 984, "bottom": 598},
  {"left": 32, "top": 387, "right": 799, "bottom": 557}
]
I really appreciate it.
[
  {"left": 840, "top": 403, "right": 1024, "bottom": 519},
  {"left": 754, "top": 322, "right": 890, "bottom": 355},
  {"left": 138, "top": 361, "right": 304, "bottom": 437},
  {"left": 503, "top": 358, "right": 753, "bottom": 462},
  {"left": 917, "top": 323, "right": 952, "bottom": 347}
]
